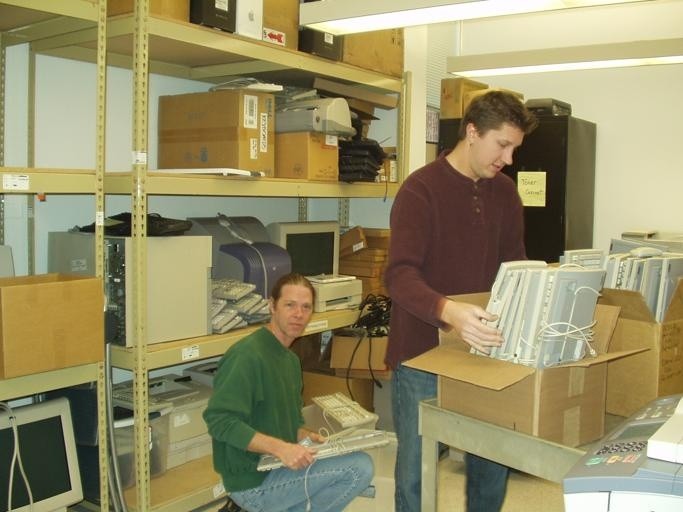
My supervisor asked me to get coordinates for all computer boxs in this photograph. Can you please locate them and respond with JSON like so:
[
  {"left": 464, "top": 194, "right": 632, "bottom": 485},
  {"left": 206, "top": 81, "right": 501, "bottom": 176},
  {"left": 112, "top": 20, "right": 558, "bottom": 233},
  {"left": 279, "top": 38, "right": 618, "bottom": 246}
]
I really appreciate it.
[
  {"left": 312, "top": 279, "right": 363, "bottom": 313},
  {"left": 104, "top": 234, "right": 214, "bottom": 348}
]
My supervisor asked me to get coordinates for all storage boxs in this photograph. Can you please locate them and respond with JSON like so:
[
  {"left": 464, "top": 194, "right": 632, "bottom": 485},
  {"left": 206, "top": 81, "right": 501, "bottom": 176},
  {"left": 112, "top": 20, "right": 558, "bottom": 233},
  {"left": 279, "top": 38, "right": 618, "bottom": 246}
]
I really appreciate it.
[
  {"left": 109, "top": 2, "right": 404, "bottom": 80},
  {"left": 440, "top": 76, "right": 523, "bottom": 121},
  {"left": 300, "top": 333, "right": 391, "bottom": 413},
  {"left": 598, "top": 276, "right": 683, "bottom": 423},
  {"left": 0, "top": 269, "right": 106, "bottom": 380},
  {"left": 156, "top": 90, "right": 275, "bottom": 180},
  {"left": 400, "top": 291, "right": 621, "bottom": 446},
  {"left": 274, "top": 132, "right": 339, "bottom": 183}
]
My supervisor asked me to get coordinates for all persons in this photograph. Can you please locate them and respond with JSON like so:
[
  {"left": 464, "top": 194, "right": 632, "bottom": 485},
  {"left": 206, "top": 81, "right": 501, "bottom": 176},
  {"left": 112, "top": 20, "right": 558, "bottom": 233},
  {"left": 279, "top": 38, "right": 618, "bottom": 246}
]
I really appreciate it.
[
  {"left": 200, "top": 273, "right": 376, "bottom": 512},
  {"left": 381, "top": 90, "right": 530, "bottom": 512}
]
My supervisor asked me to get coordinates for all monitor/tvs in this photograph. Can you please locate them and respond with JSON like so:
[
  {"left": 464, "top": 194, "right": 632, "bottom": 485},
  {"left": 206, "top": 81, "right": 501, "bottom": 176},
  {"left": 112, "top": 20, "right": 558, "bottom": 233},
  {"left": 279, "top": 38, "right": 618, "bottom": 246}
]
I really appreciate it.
[
  {"left": 265, "top": 220, "right": 340, "bottom": 278},
  {"left": 0, "top": 395, "right": 85, "bottom": 512},
  {"left": 184, "top": 214, "right": 292, "bottom": 299}
]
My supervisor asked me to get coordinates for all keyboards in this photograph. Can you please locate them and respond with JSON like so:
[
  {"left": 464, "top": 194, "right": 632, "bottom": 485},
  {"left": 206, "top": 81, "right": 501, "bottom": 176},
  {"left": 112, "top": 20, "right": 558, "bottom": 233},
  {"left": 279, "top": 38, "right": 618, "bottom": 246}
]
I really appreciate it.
[
  {"left": 256, "top": 428, "right": 390, "bottom": 477},
  {"left": 310, "top": 391, "right": 375, "bottom": 429},
  {"left": 469, "top": 247, "right": 683, "bottom": 368}
]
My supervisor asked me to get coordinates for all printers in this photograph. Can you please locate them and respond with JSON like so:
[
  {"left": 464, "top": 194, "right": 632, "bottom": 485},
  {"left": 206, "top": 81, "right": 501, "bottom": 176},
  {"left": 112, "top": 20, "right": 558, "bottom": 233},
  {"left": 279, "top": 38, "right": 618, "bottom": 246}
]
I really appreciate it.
[{"left": 560, "top": 392, "right": 683, "bottom": 512}]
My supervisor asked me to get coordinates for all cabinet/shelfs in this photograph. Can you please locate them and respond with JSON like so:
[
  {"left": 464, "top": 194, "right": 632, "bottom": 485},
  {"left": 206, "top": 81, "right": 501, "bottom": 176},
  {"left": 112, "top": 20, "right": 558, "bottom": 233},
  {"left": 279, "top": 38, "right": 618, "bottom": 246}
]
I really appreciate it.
[
  {"left": 416, "top": 395, "right": 627, "bottom": 512},
  {"left": 27, "top": 0, "right": 412, "bottom": 512},
  {"left": 1, "top": 1, "right": 108, "bottom": 512},
  {"left": 438, "top": 117, "right": 597, "bottom": 261}
]
